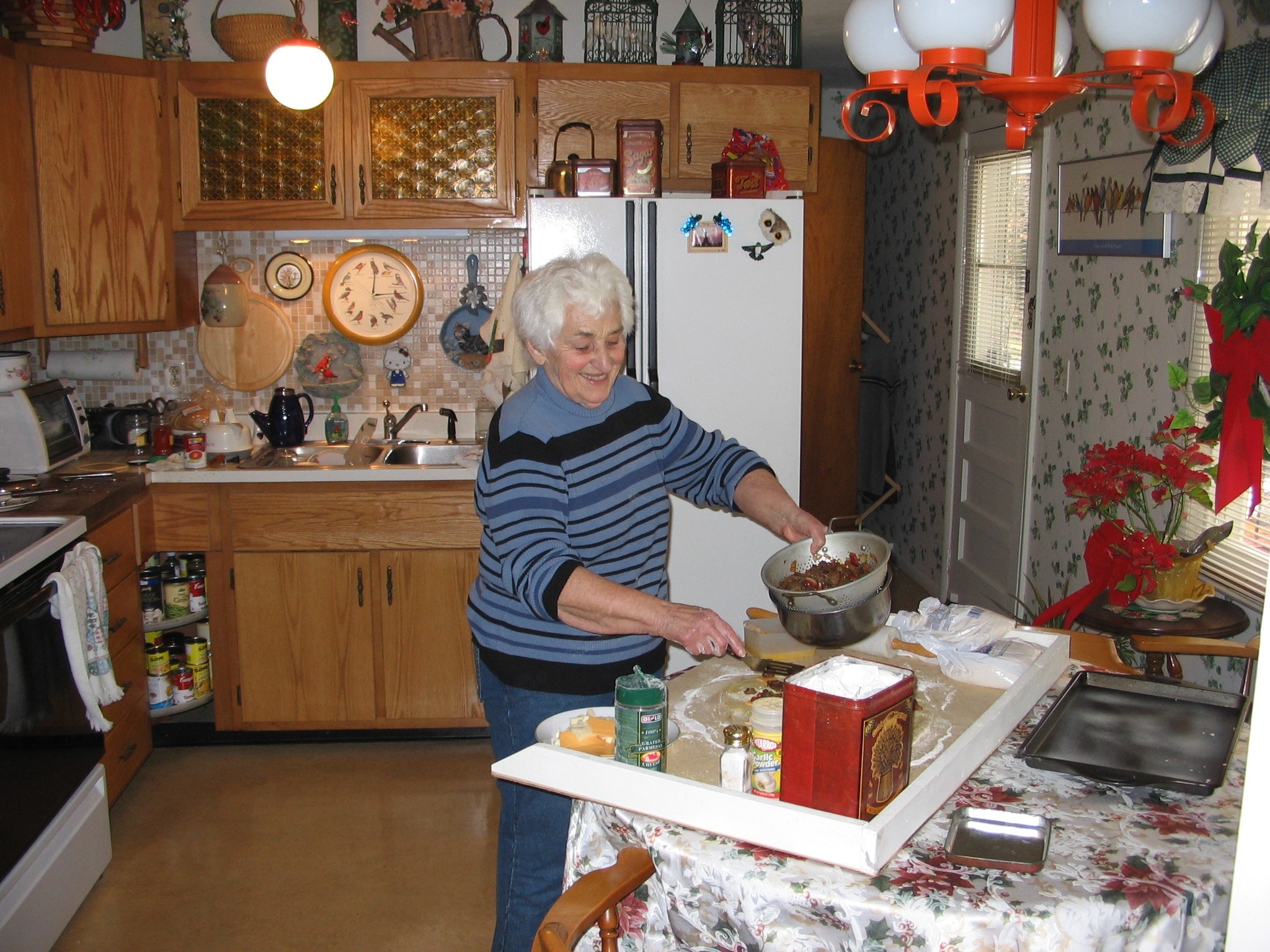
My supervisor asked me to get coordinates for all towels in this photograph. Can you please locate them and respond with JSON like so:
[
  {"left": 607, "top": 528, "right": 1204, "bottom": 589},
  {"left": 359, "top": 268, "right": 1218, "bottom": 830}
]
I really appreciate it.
[
  {"left": 479, "top": 249, "right": 532, "bottom": 416},
  {"left": 42, "top": 541, "right": 125, "bottom": 731}
]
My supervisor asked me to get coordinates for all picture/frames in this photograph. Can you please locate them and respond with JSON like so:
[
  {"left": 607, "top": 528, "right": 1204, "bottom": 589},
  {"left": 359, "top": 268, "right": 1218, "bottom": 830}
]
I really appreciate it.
[
  {"left": 688, "top": 221, "right": 729, "bottom": 254},
  {"left": 1056, "top": 148, "right": 1171, "bottom": 259}
]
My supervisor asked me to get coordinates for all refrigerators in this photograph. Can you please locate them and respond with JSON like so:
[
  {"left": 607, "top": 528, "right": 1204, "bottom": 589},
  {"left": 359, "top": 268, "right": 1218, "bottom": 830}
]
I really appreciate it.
[{"left": 525, "top": 187, "right": 805, "bottom": 677}]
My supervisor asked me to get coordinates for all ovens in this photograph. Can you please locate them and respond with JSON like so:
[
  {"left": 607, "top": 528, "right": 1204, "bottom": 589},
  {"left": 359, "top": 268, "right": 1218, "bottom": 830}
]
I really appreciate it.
[{"left": 1, "top": 538, "right": 106, "bottom": 887}]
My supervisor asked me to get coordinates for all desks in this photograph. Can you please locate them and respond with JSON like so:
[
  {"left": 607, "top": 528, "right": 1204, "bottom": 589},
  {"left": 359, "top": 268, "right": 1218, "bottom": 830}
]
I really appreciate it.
[{"left": 1061, "top": 590, "right": 1250, "bottom": 680}]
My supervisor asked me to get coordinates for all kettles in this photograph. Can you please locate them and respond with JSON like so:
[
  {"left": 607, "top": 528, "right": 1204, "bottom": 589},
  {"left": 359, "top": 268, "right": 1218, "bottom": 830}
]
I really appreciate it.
[{"left": 545, "top": 121, "right": 599, "bottom": 198}]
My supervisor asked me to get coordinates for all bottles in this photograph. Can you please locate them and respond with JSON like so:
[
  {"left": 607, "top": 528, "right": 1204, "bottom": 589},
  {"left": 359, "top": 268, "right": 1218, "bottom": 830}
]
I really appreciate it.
[
  {"left": 126, "top": 413, "right": 150, "bottom": 456},
  {"left": 719, "top": 724, "right": 752, "bottom": 794}
]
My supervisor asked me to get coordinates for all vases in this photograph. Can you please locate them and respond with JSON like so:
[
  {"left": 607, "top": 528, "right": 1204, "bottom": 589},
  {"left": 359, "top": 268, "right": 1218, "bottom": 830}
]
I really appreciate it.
[{"left": 1090, "top": 518, "right": 1233, "bottom": 600}]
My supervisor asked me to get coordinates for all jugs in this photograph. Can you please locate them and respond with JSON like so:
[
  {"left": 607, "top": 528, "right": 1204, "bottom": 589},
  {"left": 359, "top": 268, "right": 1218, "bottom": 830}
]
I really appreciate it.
[{"left": 254, "top": 387, "right": 313, "bottom": 448}]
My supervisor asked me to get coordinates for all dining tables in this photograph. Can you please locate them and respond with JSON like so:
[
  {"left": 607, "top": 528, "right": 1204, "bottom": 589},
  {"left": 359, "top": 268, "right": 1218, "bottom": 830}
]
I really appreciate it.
[{"left": 561, "top": 657, "right": 1251, "bottom": 951}]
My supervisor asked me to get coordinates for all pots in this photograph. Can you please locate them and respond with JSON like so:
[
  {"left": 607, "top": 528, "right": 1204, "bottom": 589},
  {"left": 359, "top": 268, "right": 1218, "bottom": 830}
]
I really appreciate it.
[{"left": 761, "top": 514, "right": 893, "bottom": 649}]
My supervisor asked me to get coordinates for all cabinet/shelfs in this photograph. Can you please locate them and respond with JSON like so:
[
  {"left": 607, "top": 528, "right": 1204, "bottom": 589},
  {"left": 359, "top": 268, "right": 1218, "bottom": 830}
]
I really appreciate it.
[
  {"left": 87, "top": 480, "right": 216, "bottom": 810},
  {"left": 536, "top": 63, "right": 819, "bottom": 196},
  {"left": 165, "top": 61, "right": 527, "bottom": 231},
  {"left": 226, "top": 487, "right": 494, "bottom": 731},
  {"left": 0, "top": 45, "right": 175, "bottom": 344}
]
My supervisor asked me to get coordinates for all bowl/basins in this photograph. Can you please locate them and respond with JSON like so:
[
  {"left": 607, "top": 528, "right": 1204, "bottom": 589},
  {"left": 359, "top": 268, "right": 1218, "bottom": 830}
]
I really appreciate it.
[
  {"left": 199, "top": 421, "right": 253, "bottom": 453},
  {"left": 0, "top": 350, "right": 33, "bottom": 391}
]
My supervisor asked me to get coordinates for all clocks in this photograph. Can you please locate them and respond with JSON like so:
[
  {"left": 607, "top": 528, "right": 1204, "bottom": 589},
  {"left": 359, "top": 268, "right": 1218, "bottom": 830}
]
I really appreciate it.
[{"left": 322, "top": 244, "right": 425, "bottom": 347}]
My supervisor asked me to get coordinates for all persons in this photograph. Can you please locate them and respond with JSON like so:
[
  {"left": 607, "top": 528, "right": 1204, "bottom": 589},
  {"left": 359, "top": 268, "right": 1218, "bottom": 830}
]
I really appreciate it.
[{"left": 466, "top": 250, "right": 836, "bottom": 952}]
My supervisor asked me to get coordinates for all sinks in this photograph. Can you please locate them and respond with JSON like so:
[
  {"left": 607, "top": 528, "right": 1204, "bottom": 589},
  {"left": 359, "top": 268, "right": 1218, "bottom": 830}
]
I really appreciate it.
[
  {"left": 241, "top": 438, "right": 390, "bottom": 476},
  {"left": 376, "top": 434, "right": 486, "bottom": 472}
]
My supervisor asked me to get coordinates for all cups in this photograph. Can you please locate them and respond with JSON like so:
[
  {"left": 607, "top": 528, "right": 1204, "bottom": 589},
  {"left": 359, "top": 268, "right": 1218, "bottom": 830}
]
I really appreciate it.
[{"left": 273, "top": 449, "right": 298, "bottom": 467}]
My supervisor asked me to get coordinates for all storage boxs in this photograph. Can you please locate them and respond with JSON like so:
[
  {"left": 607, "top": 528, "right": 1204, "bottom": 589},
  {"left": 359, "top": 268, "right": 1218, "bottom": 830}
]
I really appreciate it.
[
  {"left": 571, "top": 158, "right": 616, "bottom": 198},
  {"left": 615, "top": 118, "right": 663, "bottom": 197},
  {"left": 709, "top": 162, "right": 766, "bottom": 199},
  {"left": 780, "top": 656, "right": 916, "bottom": 822}
]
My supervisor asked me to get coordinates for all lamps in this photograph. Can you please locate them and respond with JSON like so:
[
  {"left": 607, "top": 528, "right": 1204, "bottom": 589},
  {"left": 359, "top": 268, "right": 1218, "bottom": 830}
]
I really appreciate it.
[
  {"left": 840, "top": 1, "right": 1225, "bottom": 151},
  {"left": 265, "top": 0, "right": 334, "bottom": 110}
]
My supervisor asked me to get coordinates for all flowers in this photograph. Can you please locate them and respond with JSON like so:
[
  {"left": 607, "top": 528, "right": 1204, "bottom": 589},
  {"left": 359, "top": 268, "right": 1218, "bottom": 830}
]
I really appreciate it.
[
  {"left": 1063, "top": 415, "right": 1219, "bottom": 594},
  {"left": 1182, "top": 286, "right": 1209, "bottom": 306}
]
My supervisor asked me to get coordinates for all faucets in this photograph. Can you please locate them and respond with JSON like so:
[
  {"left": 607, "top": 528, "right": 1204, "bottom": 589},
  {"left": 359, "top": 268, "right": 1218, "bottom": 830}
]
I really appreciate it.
[{"left": 379, "top": 391, "right": 428, "bottom": 446}]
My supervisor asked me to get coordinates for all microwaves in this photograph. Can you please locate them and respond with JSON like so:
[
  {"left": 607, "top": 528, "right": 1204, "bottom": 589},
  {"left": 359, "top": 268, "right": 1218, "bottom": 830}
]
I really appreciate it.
[{"left": 0, "top": 377, "right": 94, "bottom": 471}]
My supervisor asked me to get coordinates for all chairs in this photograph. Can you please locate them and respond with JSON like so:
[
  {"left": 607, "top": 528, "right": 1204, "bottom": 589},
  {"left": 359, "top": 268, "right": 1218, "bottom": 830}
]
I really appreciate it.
[
  {"left": 1019, "top": 626, "right": 1146, "bottom": 677},
  {"left": 530, "top": 846, "right": 656, "bottom": 952},
  {"left": 1130, "top": 633, "right": 1260, "bottom": 660}
]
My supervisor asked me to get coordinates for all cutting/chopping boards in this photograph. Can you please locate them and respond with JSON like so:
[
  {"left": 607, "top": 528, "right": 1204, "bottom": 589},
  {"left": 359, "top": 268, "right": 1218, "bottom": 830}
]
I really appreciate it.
[{"left": 198, "top": 256, "right": 295, "bottom": 393}]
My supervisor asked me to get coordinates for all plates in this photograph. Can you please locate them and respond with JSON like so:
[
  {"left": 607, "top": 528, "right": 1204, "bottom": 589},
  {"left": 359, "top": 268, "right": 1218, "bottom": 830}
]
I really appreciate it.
[
  {"left": 307, "top": 449, "right": 346, "bottom": 465},
  {"left": 0, "top": 494, "right": 40, "bottom": 513},
  {"left": 264, "top": 252, "right": 313, "bottom": 300},
  {"left": 535, "top": 707, "right": 680, "bottom": 760}
]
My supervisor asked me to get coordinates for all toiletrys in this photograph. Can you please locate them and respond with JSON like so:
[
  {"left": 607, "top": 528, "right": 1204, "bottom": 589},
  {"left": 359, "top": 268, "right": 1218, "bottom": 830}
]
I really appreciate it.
[{"left": 323, "top": 392, "right": 351, "bottom": 447}]
